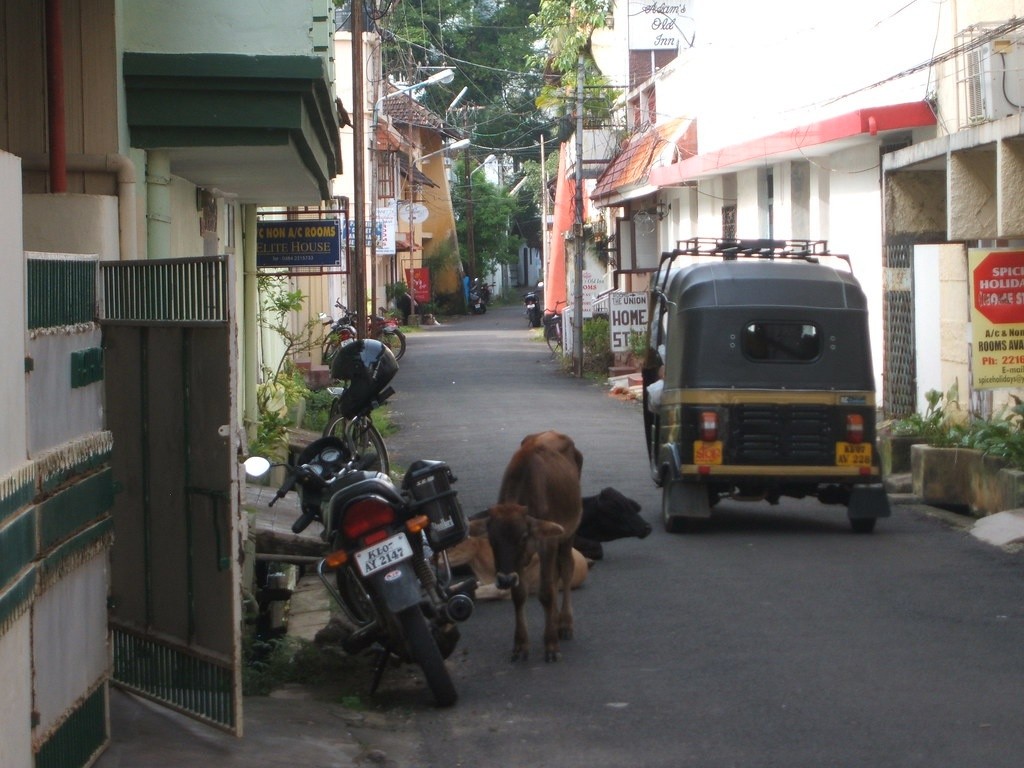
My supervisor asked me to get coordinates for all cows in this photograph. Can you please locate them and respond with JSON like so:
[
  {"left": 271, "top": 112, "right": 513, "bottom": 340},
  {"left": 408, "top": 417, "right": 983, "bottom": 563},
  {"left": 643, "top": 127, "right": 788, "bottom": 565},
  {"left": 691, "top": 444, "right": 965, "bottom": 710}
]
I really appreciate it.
[{"left": 445, "top": 428, "right": 652, "bottom": 664}]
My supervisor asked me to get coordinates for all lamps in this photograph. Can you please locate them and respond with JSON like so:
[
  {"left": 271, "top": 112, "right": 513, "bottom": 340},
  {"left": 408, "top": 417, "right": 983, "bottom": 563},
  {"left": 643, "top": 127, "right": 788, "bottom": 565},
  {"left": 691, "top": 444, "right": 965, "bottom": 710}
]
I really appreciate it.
[
  {"left": 589, "top": 213, "right": 616, "bottom": 251},
  {"left": 656, "top": 199, "right": 671, "bottom": 221}
]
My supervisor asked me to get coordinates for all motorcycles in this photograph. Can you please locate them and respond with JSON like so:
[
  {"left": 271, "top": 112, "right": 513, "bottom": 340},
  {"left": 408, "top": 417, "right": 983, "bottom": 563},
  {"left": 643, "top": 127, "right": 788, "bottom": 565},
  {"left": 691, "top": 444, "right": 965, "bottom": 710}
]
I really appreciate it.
[
  {"left": 244, "top": 385, "right": 478, "bottom": 710},
  {"left": 521, "top": 281, "right": 546, "bottom": 330},
  {"left": 637, "top": 236, "right": 891, "bottom": 529}
]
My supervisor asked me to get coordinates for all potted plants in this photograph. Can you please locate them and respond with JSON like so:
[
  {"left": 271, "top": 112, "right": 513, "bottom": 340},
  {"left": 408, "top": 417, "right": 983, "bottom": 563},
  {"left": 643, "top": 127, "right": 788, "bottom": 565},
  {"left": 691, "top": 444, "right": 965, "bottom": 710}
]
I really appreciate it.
[{"left": 880, "top": 378, "right": 1024, "bottom": 513}]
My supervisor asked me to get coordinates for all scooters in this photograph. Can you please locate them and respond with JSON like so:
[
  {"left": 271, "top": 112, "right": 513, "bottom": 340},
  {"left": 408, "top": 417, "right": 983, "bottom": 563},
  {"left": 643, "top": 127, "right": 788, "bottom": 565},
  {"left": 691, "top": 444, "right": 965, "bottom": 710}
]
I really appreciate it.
[{"left": 469, "top": 278, "right": 497, "bottom": 313}]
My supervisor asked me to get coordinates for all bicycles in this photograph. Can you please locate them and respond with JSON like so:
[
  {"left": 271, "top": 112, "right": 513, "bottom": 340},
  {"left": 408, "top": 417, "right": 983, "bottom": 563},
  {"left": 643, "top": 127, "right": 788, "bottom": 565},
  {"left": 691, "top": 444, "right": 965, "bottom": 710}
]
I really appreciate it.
[
  {"left": 539, "top": 300, "right": 566, "bottom": 357},
  {"left": 322, "top": 298, "right": 407, "bottom": 364}
]
